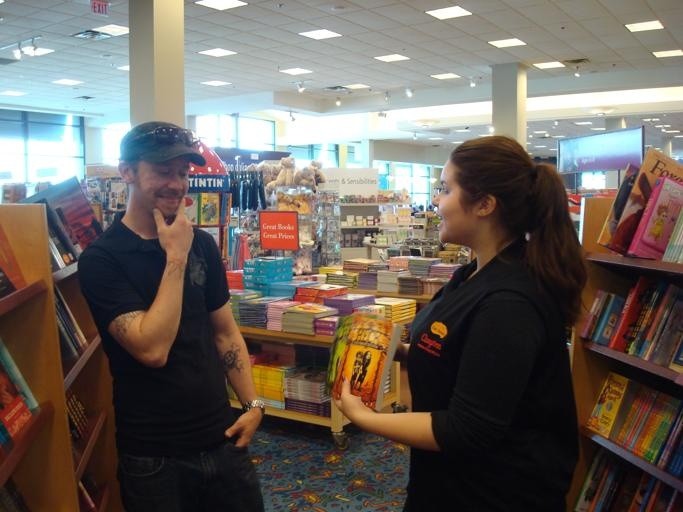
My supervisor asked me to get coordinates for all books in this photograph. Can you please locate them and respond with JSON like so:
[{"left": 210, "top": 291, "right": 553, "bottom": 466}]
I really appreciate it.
[
  {"left": 224, "top": 252, "right": 470, "bottom": 421},
  {"left": 561, "top": 145, "right": 682, "bottom": 512},
  {"left": 0, "top": 176, "right": 104, "bottom": 510},
  {"left": 371, "top": 207, "right": 469, "bottom": 251},
  {"left": 104, "top": 175, "right": 235, "bottom": 264}
]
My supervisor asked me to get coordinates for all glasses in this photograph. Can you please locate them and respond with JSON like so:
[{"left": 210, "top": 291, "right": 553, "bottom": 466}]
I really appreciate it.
[
  {"left": 436, "top": 184, "right": 451, "bottom": 197},
  {"left": 126, "top": 127, "right": 193, "bottom": 147}
]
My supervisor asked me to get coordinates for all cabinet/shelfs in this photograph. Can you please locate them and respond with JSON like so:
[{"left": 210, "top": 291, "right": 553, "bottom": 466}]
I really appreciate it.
[
  {"left": 570, "top": 197, "right": 682, "bottom": 512},
  {"left": 339, "top": 201, "right": 393, "bottom": 249},
  {"left": 0, "top": 200, "right": 121, "bottom": 512},
  {"left": 218, "top": 292, "right": 434, "bottom": 435}
]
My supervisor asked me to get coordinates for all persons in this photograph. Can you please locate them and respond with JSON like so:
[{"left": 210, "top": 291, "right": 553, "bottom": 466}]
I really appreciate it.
[
  {"left": 76, "top": 120, "right": 270, "bottom": 512},
  {"left": 332, "top": 131, "right": 590, "bottom": 512}
]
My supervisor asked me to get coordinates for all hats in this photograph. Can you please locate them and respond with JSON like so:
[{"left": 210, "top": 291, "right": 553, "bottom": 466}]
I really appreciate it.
[{"left": 119, "top": 121, "right": 207, "bottom": 167}]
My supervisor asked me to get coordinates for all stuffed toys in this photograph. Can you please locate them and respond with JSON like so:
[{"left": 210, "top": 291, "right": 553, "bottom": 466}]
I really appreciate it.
[{"left": 245, "top": 155, "right": 328, "bottom": 214}]
